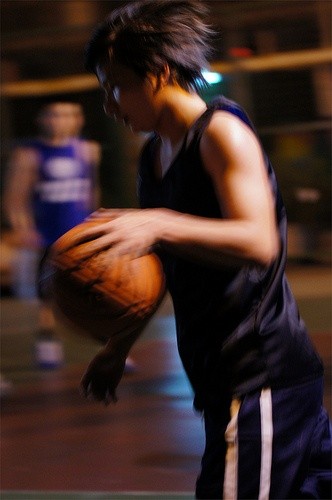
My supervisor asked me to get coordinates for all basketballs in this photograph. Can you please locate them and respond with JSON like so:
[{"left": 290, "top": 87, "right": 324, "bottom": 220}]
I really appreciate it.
[{"left": 40, "top": 222, "right": 165, "bottom": 343}]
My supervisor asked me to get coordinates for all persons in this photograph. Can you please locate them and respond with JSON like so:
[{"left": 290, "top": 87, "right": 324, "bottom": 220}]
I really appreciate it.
[
  {"left": 0, "top": 95, "right": 142, "bottom": 379},
  {"left": 77, "top": 0, "right": 332, "bottom": 499}
]
